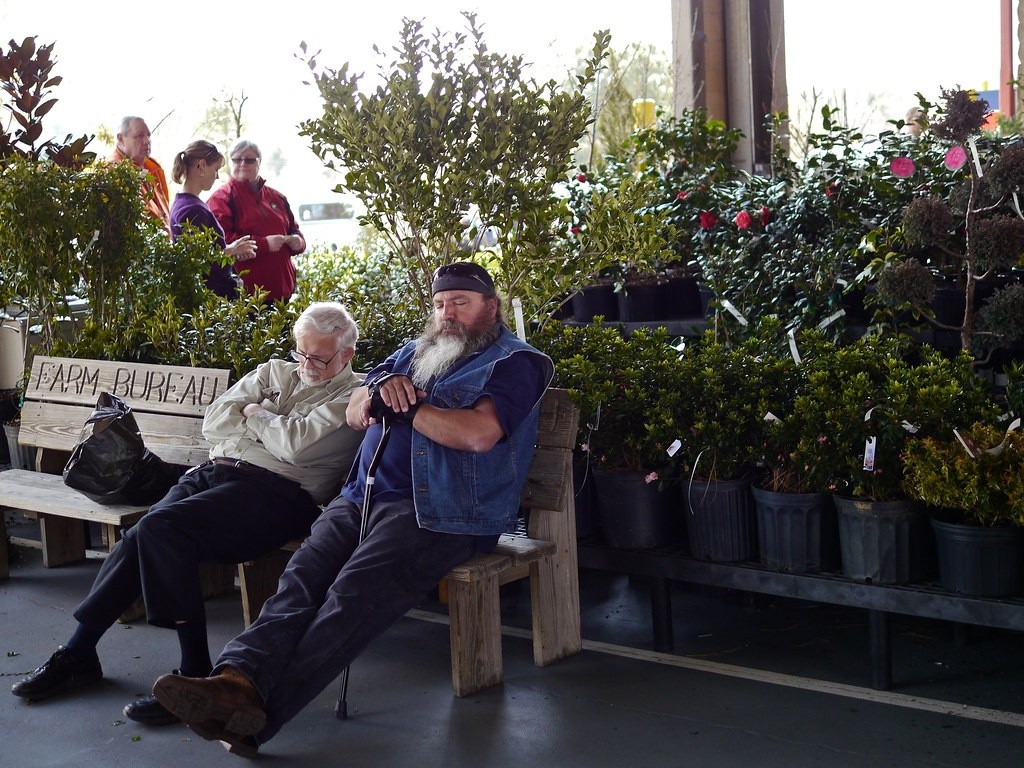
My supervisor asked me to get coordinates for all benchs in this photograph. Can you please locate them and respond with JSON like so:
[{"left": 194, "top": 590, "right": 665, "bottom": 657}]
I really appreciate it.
[
  {"left": 237, "top": 373, "right": 582, "bottom": 699},
  {"left": 0, "top": 355, "right": 230, "bottom": 624}
]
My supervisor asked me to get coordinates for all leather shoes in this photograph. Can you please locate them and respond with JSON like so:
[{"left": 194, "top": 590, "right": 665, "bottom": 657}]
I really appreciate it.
[
  {"left": 124, "top": 669, "right": 181, "bottom": 723},
  {"left": 12, "top": 644, "right": 104, "bottom": 698},
  {"left": 185, "top": 717, "right": 258, "bottom": 758},
  {"left": 152, "top": 666, "right": 268, "bottom": 733}
]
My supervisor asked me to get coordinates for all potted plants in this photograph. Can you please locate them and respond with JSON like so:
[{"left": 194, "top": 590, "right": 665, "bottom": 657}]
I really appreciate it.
[
  {"left": 568, "top": 90, "right": 747, "bottom": 323},
  {"left": 0, "top": 35, "right": 97, "bottom": 470},
  {"left": 521, "top": 310, "right": 1024, "bottom": 601}
]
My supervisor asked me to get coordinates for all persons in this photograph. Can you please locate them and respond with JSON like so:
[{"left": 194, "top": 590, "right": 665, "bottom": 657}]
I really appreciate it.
[
  {"left": 168, "top": 140, "right": 258, "bottom": 308},
  {"left": 109, "top": 116, "right": 171, "bottom": 244},
  {"left": 150, "top": 261, "right": 560, "bottom": 754},
  {"left": 206, "top": 140, "right": 307, "bottom": 322},
  {"left": 8, "top": 300, "right": 372, "bottom": 729}
]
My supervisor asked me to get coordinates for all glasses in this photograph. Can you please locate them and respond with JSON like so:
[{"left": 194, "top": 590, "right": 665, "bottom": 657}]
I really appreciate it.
[
  {"left": 432, "top": 264, "right": 490, "bottom": 292},
  {"left": 290, "top": 349, "right": 341, "bottom": 370},
  {"left": 197, "top": 146, "right": 218, "bottom": 169},
  {"left": 231, "top": 157, "right": 259, "bottom": 165}
]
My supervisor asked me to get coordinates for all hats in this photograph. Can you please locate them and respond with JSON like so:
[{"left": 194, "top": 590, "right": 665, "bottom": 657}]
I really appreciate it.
[{"left": 431, "top": 262, "right": 495, "bottom": 298}]
